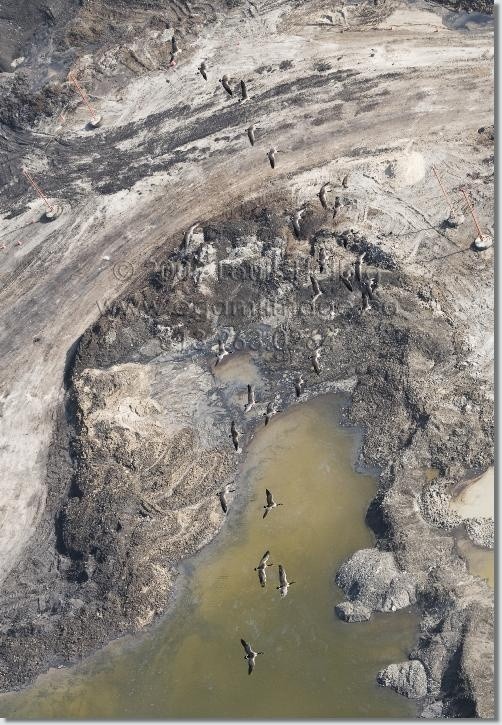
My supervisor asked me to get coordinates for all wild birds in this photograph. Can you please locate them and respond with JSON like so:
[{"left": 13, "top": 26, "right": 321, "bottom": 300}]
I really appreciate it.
[
  {"left": 215, "top": 479, "right": 237, "bottom": 515},
  {"left": 262, "top": 488, "right": 284, "bottom": 520},
  {"left": 245, "top": 120, "right": 278, "bottom": 168},
  {"left": 253, "top": 551, "right": 296, "bottom": 600},
  {"left": 294, "top": 346, "right": 324, "bottom": 398},
  {"left": 239, "top": 636, "right": 264, "bottom": 676},
  {"left": 292, "top": 173, "right": 376, "bottom": 317},
  {"left": 198, "top": 61, "right": 252, "bottom": 107},
  {"left": 167, "top": 34, "right": 189, "bottom": 66},
  {"left": 213, "top": 338, "right": 235, "bottom": 368},
  {"left": 229, "top": 384, "right": 283, "bottom": 451}
]
